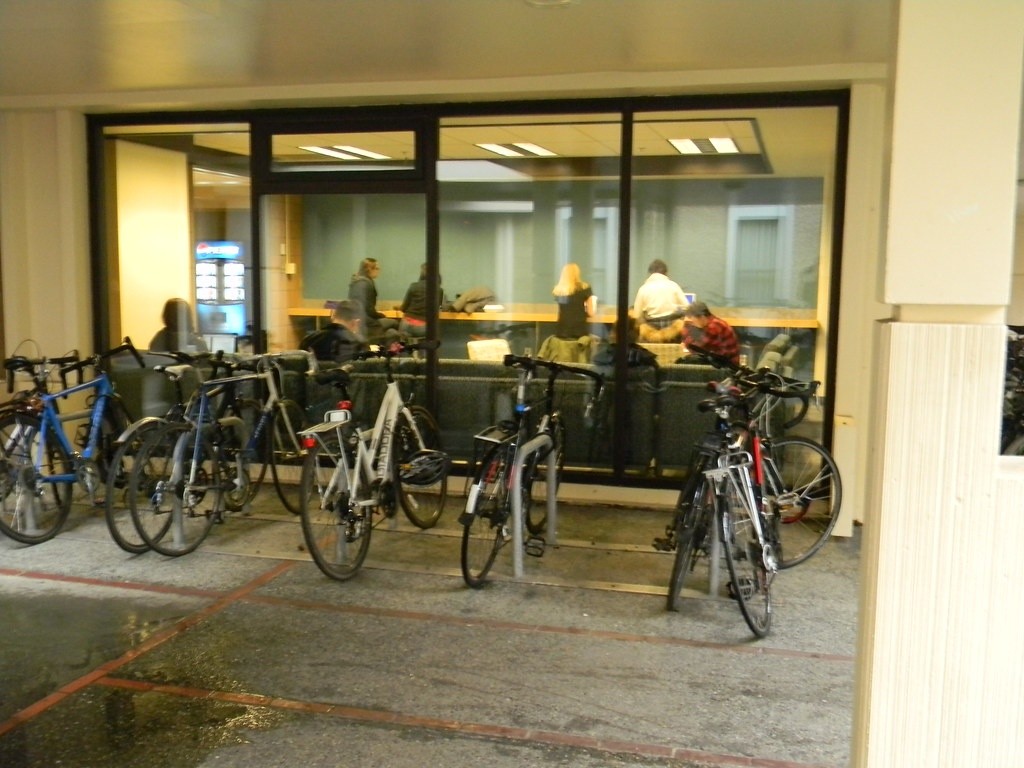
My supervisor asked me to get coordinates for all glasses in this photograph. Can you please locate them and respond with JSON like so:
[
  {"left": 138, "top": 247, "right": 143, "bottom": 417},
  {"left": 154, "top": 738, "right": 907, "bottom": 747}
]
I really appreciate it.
[{"left": 374, "top": 267, "right": 379, "bottom": 270}]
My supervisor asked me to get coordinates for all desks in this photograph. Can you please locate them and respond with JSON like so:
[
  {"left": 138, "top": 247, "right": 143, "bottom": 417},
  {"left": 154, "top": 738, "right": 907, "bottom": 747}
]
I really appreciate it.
[{"left": 287, "top": 297, "right": 819, "bottom": 347}]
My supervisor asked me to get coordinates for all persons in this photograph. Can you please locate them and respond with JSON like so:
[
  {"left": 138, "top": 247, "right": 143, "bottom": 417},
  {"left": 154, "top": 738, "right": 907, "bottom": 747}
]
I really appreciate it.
[
  {"left": 552, "top": 263, "right": 594, "bottom": 341},
  {"left": 349, "top": 257, "right": 400, "bottom": 351},
  {"left": 634, "top": 259, "right": 691, "bottom": 329},
  {"left": 149, "top": 298, "right": 210, "bottom": 354},
  {"left": 299, "top": 298, "right": 371, "bottom": 360},
  {"left": 674, "top": 300, "right": 740, "bottom": 367},
  {"left": 591, "top": 314, "right": 660, "bottom": 367},
  {"left": 398, "top": 261, "right": 444, "bottom": 359}
]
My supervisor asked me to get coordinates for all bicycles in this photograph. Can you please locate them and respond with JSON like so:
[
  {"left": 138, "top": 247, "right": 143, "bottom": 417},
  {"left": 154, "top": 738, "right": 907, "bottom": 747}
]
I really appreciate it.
[
  {"left": 0, "top": 338, "right": 154, "bottom": 545},
  {"left": 654, "top": 341, "right": 844, "bottom": 637},
  {"left": 296, "top": 335, "right": 452, "bottom": 581},
  {"left": 459, "top": 354, "right": 606, "bottom": 589},
  {"left": 101, "top": 352, "right": 315, "bottom": 559}
]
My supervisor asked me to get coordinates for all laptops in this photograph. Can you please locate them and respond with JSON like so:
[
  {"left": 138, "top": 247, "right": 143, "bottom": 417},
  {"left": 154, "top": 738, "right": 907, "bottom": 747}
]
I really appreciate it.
[{"left": 685, "top": 293, "right": 698, "bottom": 304}]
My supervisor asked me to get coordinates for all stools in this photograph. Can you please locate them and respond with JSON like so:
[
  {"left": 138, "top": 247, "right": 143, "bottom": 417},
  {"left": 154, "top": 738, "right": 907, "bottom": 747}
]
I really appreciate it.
[
  {"left": 403, "top": 330, "right": 441, "bottom": 363},
  {"left": 536, "top": 331, "right": 603, "bottom": 362}
]
